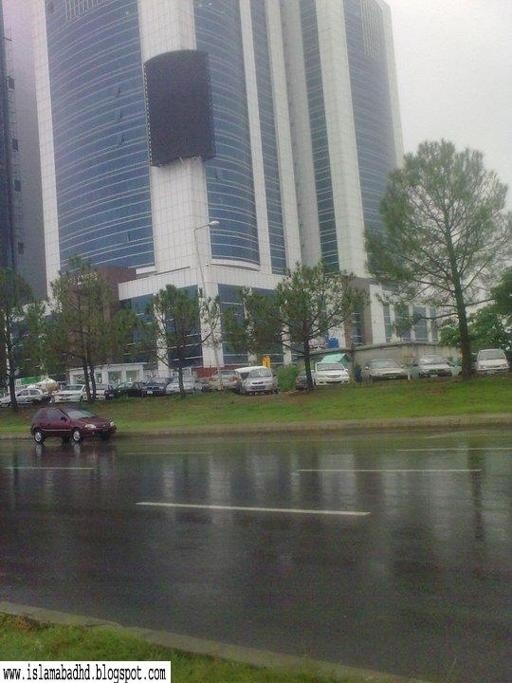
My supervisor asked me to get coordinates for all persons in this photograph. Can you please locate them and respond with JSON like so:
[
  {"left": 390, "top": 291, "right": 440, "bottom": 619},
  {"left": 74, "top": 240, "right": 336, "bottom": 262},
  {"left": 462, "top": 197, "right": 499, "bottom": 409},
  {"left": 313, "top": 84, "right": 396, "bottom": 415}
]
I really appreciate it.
[
  {"left": 109, "top": 386, "right": 113, "bottom": 400},
  {"left": 105, "top": 385, "right": 110, "bottom": 400}
]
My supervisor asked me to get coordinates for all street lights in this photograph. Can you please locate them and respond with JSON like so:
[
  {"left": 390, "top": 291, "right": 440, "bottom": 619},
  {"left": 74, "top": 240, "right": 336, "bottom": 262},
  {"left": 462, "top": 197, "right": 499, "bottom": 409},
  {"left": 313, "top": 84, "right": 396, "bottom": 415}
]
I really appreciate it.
[{"left": 192, "top": 220, "right": 225, "bottom": 393}]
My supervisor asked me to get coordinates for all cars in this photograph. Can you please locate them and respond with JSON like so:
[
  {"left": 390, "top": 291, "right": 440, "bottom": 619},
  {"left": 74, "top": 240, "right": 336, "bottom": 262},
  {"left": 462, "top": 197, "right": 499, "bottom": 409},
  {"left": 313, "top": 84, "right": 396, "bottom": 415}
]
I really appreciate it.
[
  {"left": 311, "top": 361, "right": 350, "bottom": 387},
  {"left": 410, "top": 354, "right": 452, "bottom": 380},
  {"left": 29, "top": 406, "right": 116, "bottom": 443},
  {"left": 360, "top": 357, "right": 409, "bottom": 385},
  {"left": 0, "top": 365, "right": 280, "bottom": 405},
  {"left": 295, "top": 369, "right": 315, "bottom": 391},
  {"left": 474, "top": 348, "right": 511, "bottom": 378}
]
